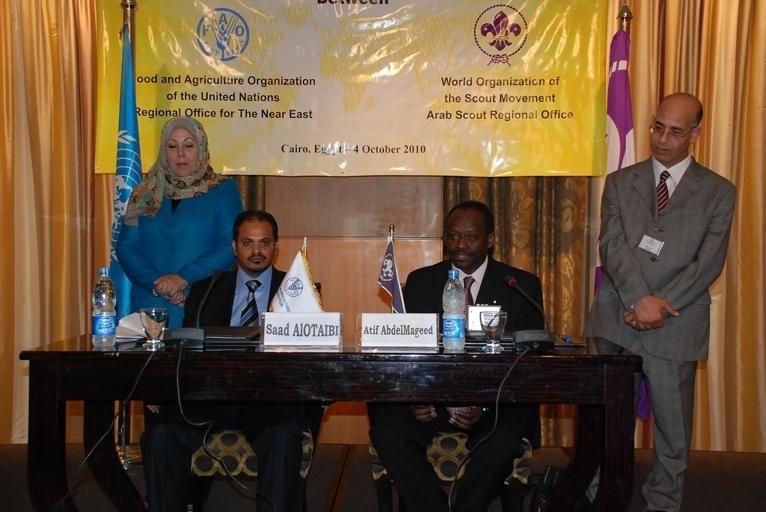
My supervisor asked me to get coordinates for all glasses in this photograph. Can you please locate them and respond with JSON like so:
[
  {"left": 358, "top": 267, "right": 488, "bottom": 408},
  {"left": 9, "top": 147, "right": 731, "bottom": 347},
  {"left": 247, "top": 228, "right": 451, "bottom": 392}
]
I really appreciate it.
[
  {"left": 236, "top": 238, "right": 278, "bottom": 250},
  {"left": 649, "top": 121, "right": 699, "bottom": 138}
]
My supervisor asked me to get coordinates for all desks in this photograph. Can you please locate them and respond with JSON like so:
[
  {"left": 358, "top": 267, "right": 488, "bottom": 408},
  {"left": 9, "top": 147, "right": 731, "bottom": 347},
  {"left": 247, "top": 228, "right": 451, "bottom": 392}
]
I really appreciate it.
[{"left": 19, "top": 332, "right": 644, "bottom": 512}]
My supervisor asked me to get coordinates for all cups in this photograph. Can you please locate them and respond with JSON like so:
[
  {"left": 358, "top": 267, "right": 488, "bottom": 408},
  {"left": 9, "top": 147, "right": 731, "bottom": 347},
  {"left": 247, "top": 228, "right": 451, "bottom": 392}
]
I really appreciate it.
[
  {"left": 480, "top": 312, "right": 508, "bottom": 353},
  {"left": 139, "top": 307, "right": 168, "bottom": 353}
]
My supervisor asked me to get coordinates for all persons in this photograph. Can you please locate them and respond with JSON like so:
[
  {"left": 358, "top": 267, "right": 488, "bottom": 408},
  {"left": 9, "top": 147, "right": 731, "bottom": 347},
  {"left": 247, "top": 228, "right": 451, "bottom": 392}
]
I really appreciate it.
[
  {"left": 115, "top": 115, "right": 244, "bottom": 432},
  {"left": 138, "top": 209, "right": 324, "bottom": 512},
  {"left": 581, "top": 92, "right": 738, "bottom": 512},
  {"left": 365, "top": 199, "right": 544, "bottom": 512}
]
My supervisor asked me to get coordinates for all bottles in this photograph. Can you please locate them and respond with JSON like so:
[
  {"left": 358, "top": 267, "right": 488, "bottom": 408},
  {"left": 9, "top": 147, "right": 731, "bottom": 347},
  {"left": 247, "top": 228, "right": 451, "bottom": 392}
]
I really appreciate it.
[
  {"left": 443, "top": 271, "right": 467, "bottom": 350},
  {"left": 92, "top": 267, "right": 117, "bottom": 347}
]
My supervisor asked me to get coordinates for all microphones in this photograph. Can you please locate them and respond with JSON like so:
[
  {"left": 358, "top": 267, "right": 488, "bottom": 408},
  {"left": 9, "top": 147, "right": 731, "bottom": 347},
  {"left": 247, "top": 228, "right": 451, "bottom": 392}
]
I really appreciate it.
[
  {"left": 504, "top": 274, "right": 556, "bottom": 352},
  {"left": 161, "top": 269, "right": 225, "bottom": 349}
]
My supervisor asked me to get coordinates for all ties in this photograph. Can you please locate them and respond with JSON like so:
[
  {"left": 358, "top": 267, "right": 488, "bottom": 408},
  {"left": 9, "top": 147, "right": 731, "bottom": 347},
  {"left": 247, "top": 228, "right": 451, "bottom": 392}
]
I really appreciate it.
[
  {"left": 442, "top": 231, "right": 491, "bottom": 244},
  {"left": 462, "top": 276, "right": 476, "bottom": 321},
  {"left": 237, "top": 281, "right": 261, "bottom": 329},
  {"left": 655, "top": 171, "right": 671, "bottom": 216}
]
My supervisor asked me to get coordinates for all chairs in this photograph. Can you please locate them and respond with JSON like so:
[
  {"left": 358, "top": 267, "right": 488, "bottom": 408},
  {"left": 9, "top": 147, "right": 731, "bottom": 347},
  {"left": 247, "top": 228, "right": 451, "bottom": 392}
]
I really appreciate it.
[
  {"left": 192, "top": 281, "right": 320, "bottom": 512},
  {"left": 368, "top": 423, "right": 534, "bottom": 512}
]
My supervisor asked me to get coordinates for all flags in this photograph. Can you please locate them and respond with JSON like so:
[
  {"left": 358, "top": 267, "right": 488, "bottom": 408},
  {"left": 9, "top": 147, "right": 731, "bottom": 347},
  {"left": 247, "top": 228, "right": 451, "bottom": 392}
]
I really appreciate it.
[
  {"left": 108, "top": 2, "right": 143, "bottom": 444},
  {"left": 594, "top": 4, "right": 652, "bottom": 422},
  {"left": 376, "top": 225, "right": 407, "bottom": 313},
  {"left": 269, "top": 236, "right": 323, "bottom": 313}
]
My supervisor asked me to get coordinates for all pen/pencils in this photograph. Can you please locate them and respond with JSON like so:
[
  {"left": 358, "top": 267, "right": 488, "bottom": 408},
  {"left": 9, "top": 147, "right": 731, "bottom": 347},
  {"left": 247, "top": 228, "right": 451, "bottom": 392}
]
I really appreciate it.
[{"left": 559, "top": 334, "right": 572, "bottom": 343}]
